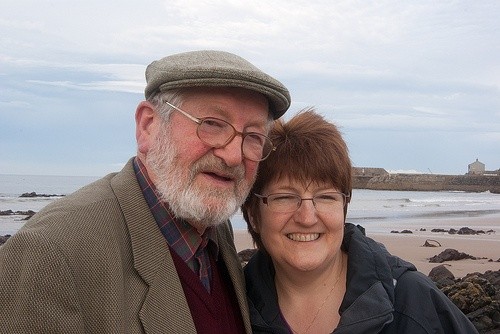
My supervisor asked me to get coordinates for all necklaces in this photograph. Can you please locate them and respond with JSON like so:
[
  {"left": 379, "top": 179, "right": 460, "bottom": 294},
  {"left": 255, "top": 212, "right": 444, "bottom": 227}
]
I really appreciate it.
[{"left": 277, "top": 259, "right": 344, "bottom": 334}]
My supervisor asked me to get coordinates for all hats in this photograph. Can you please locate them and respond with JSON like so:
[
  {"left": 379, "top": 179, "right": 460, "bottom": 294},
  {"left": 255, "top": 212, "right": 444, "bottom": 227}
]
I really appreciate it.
[{"left": 144, "top": 51, "right": 291, "bottom": 120}]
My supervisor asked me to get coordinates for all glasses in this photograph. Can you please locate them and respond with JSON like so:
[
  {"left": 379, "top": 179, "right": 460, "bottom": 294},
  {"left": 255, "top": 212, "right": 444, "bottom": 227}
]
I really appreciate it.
[
  {"left": 166, "top": 101, "right": 276, "bottom": 161},
  {"left": 253, "top": 192, "right": 350, "bottom": 213}
]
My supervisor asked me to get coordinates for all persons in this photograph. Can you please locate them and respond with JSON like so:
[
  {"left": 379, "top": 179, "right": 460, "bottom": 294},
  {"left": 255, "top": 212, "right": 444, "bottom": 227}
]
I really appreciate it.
[
  {"left": 0, "top": 50, "right": 291, "bottom": 333},
  {"left": 243, "top": 108, "right": 478, "bottom": 334}
]
